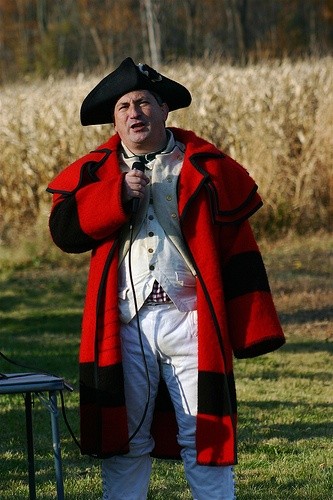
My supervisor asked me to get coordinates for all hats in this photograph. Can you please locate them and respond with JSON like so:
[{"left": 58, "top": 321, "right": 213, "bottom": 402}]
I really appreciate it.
[{"left": 80, "top": 56, "right": 191, "bottom": 126}]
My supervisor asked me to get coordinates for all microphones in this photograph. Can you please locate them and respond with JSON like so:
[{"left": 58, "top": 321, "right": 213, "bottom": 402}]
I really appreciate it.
[{"left": 130, "top": 162, "right": 145, "bottom": 228}]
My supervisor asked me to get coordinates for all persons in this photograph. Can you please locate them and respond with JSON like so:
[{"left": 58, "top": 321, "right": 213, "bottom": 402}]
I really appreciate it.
[{"left": 45, "top": 57, "right": 285, "bottom": 500}]
